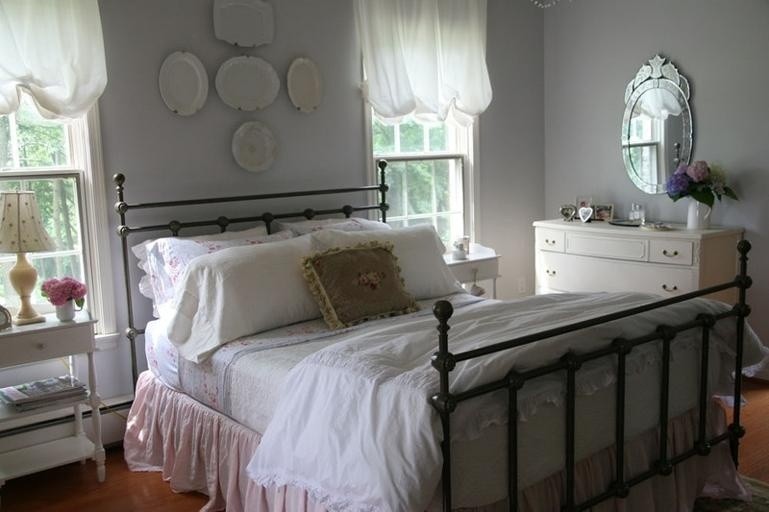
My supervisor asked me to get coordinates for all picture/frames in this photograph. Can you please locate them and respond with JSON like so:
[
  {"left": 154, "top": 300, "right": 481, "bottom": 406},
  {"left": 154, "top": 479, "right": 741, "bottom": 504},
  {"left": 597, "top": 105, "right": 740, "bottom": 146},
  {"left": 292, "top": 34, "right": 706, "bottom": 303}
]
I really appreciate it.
[
  {"left": 559, "top": 206, "right": 576, "bottom": 221},
  {"left": 575, "top": 195, "right": 592, "bottom": 218},
  {"left": 579, "top": 207, "right": 593, "bottom": 223},
  {"left": 591, "top": 204, "right": 614, "bottom": 221}
]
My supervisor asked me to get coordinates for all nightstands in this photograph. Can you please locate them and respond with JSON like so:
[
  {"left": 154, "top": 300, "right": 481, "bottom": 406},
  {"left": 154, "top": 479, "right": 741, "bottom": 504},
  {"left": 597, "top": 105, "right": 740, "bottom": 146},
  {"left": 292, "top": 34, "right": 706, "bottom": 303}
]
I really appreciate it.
[
  {"left": 0, "top": 310, "right": 107, "bottom": 483},
  {"left": 442, "top": 248, "right": 502, "bottom": 299}
]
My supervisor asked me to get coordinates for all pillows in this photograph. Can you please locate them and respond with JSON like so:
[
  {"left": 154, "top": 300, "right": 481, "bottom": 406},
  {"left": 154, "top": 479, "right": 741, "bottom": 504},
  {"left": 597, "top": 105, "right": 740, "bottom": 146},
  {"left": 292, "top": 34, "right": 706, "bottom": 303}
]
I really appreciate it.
[
  {"left": 310, "top": 222, "right": 464, "bottom": 300},
  {"left": 278, "top": 217, "right": 392, "bottom": 236},
  {"left": 147, "top": 230, "right": 303, "bottom": 317},
  {"left": 133, "top": 226, "right": 269, "bottom": 317},
  {"left": 165, "top": 234, "right": 323, "bottom": 362},
  {"left": 300, "top": 241, "right": 424, "bottom": 331}
]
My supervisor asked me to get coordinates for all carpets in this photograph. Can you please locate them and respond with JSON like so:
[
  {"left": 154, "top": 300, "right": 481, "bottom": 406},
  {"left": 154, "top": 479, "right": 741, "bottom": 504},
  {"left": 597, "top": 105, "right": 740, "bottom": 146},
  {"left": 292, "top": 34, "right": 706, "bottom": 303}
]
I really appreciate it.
[{"left": 694, "top": 474, "right": 769, "bottom": 511}]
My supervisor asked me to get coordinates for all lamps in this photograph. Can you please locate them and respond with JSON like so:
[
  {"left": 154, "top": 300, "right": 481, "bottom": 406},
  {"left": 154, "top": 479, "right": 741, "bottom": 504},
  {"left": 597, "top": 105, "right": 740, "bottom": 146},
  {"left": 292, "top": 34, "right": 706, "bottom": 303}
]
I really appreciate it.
[{"left": 1, "top": 191, "right": 57, "bottom": 326}]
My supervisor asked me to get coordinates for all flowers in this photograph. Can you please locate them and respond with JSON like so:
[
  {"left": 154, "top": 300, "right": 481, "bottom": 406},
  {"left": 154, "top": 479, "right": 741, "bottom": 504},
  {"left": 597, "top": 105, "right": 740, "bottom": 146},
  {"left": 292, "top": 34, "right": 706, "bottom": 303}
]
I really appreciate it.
[
  {"left": 666, "top": 161, "right": 739, "bottom": 210},
  {"left": 41, "top": 278, "right": 86, "bottom": 309}
]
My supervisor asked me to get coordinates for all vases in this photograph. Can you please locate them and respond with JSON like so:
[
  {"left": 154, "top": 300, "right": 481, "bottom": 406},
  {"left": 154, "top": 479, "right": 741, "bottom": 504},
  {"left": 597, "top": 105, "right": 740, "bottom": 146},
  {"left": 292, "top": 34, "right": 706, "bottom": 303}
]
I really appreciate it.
[
  {"left": 56, "top": 300, "right": 83, "bottom": 322},
  {"left": 687, "top": 198, "right": 711, "bottom": 229}
]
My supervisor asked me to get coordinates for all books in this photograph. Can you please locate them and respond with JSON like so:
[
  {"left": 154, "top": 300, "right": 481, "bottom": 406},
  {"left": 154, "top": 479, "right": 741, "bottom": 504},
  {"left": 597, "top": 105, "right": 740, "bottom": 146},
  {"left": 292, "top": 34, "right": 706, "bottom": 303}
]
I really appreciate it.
[{"left": 0, "top": 374, "right": 84, "bottom": 414}]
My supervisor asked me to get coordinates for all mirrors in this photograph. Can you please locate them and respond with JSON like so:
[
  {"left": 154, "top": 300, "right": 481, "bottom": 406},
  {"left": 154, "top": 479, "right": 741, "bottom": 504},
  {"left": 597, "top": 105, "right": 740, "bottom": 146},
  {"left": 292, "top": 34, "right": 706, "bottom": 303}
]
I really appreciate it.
[{"left": 620, "top": 54, "right": 693, "bottom": 195}]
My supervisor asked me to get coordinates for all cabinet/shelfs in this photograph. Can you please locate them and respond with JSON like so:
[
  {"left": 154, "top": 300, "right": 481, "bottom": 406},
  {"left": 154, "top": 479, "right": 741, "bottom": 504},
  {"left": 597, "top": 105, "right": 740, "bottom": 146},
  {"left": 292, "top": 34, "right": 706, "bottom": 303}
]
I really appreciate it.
[{"left": 532, "top": 219, "right": 745, "bottom": 305}]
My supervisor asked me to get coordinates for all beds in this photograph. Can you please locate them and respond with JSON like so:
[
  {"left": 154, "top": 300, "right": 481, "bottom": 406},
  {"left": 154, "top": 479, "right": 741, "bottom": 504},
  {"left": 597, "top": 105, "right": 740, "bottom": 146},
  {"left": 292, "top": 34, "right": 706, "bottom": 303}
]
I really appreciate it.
[{"left": 115, "top": 170, "right": 752, "bottom": 512}]
